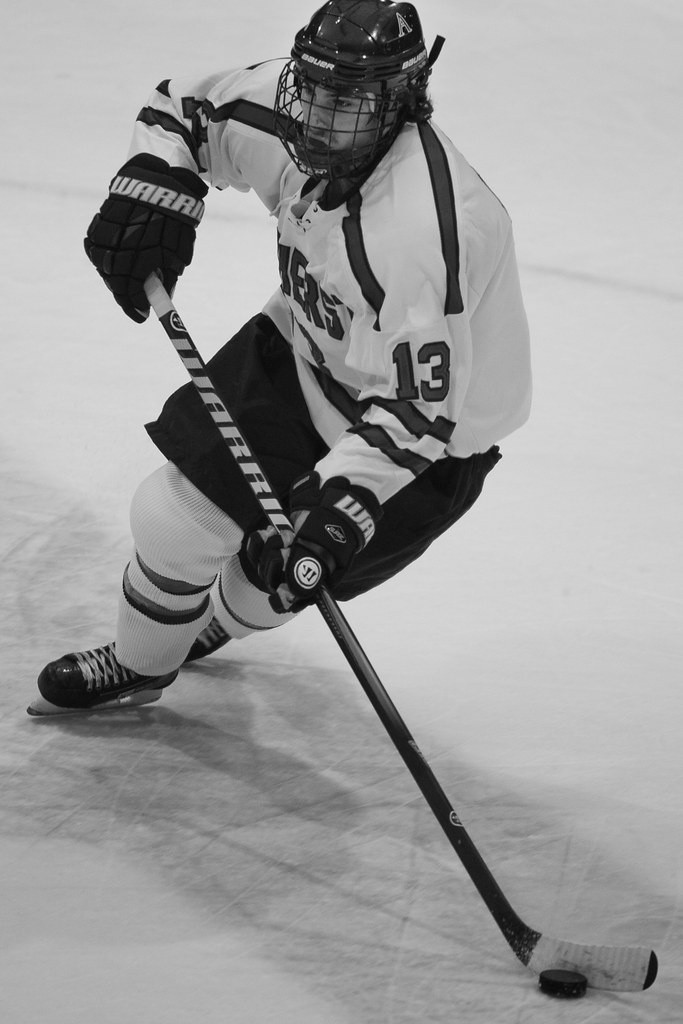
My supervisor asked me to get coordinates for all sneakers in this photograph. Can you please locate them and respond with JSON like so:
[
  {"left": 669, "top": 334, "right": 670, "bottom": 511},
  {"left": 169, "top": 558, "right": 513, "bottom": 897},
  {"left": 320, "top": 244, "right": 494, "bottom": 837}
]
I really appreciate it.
[
  {"left": 181, "top": 616, "right": 233, "bottom": 662},
  {"left": 26, "top": 641, "right": 180, "bottom": 717}
]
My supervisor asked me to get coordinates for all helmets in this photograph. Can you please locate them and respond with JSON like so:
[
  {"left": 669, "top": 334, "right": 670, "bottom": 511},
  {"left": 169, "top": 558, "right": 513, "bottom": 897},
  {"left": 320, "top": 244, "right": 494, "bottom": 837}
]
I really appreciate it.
[{"left": 291, "top": 0, "right": 433, "bottom": 178}]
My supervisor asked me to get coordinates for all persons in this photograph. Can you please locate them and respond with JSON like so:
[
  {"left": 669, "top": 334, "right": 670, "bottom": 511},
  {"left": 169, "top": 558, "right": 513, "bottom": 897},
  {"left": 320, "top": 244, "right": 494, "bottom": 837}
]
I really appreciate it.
[{"left": 35, "top": 1, "right": 533, "bottom": 713}]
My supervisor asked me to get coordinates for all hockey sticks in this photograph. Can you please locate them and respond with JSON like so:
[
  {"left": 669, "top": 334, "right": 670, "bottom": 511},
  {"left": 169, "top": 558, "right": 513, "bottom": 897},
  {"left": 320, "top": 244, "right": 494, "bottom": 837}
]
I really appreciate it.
[{"left": 137, "top": 265, "right": 663, "bottom": 995}]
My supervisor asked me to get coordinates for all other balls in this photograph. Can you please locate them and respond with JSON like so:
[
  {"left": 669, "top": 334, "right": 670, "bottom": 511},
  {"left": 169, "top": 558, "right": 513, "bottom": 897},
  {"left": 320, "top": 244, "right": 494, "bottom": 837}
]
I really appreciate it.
[{"left": 538, "top": 968, "right": 590, "bottom": 1001}]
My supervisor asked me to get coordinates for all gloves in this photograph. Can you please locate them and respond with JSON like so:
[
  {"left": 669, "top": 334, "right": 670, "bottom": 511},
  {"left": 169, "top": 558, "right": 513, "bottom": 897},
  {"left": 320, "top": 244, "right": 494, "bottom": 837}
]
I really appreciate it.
[
  {"left": 244, "top": 468, "right": 386, "bottom": 616},
  {"left": 83, "top": 151, "right": 209, "bottom": 324}
]
